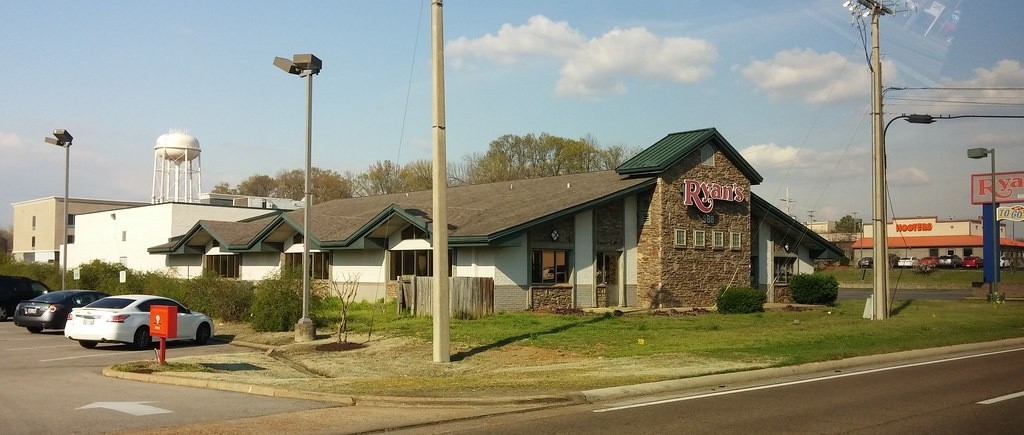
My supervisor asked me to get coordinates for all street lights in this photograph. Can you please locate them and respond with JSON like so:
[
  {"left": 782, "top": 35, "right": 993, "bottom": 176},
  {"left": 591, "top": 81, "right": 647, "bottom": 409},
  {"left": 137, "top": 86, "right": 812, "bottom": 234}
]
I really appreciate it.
[
  {"left": 967, "top": 146, "right": 1006, "bottom": 304},
  {"left": 44, "top": 128, "right": 73, "bottom": 291},
  {"left": 272, "top": 54, "right": 323, "bottom": 342}
]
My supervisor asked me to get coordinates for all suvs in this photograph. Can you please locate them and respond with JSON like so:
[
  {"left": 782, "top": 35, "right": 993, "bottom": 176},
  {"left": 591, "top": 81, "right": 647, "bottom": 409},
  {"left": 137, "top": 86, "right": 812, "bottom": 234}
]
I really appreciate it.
[{"left": 939, "top": 255, "right": 962, "bottom": 269}]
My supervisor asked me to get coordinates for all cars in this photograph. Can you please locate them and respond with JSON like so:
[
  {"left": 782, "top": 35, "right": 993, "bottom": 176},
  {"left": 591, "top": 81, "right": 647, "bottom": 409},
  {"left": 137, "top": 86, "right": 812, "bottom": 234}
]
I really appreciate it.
[
  {"left": 897, "top": 256, "right": 919, "bottom": 269},
  {"left": 888, "top": 253, "right": 901, "bottom": 269},
  {"left": 999, "top": 255, "right": 1014, "bottom": 268},
  {"left": 62, "top": 293, "right": 215, "bottom": 351},
  {"left": 858, "top": 256, "right": 873, "bottom": 268},
  {"left": 0, "top": 275, "right": 52, "bottom": 323},
  {"left": 919, "top": 257, "right": 939, "bottom": 269},
  {"left": 13, "top": 289, "right": 110, "bottom": 333},
  {"left": 961, "top": 256, "right": 983, "bottom": 269}
]
